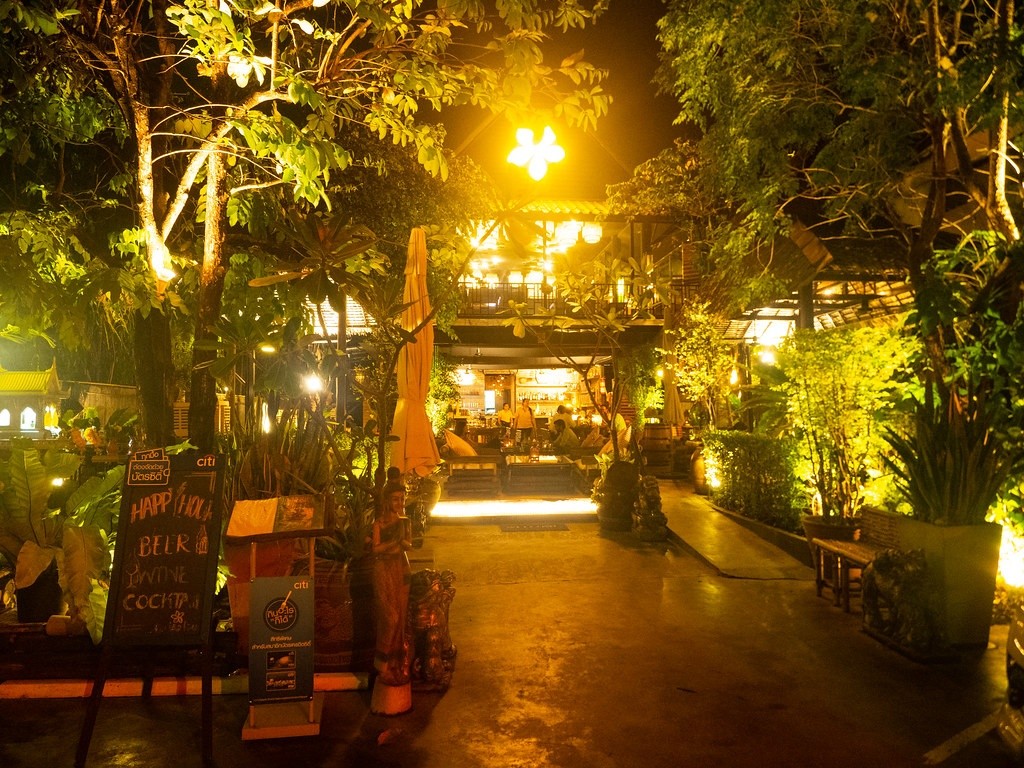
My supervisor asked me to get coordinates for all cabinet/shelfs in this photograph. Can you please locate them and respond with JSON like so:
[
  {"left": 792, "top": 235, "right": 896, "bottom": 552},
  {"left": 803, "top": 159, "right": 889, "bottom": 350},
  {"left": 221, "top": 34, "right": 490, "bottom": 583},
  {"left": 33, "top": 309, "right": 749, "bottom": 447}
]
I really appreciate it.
[{"left": 516, "top": 384, "right": 571, "bottom": 403}]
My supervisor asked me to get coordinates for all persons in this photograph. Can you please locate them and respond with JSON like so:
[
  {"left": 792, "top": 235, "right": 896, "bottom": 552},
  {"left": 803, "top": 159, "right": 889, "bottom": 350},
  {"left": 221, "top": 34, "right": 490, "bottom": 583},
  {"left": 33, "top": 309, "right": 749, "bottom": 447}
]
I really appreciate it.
[
  {"left": 69, "top": 417, "right": 136, "bottom": 457},
  {"left": 372, "top": 466, "right": 414, "bottom": 684},
  {"left": 455, "top": 399, "right": 627, "bottom": 455}
]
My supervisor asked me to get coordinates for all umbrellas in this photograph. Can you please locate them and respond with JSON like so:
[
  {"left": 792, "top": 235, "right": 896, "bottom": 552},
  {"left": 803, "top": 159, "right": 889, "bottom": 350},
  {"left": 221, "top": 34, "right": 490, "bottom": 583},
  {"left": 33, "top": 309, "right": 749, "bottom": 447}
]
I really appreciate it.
[{"left": 390, "top": 228, "right": 440, "bottom": 479}]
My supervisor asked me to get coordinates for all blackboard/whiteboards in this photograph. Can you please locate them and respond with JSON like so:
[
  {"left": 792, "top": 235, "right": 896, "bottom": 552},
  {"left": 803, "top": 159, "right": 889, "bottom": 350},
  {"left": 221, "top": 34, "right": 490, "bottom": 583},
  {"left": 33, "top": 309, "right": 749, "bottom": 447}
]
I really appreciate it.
[{"left": 100, "top": 452, "right": 229, "bottom": 650}]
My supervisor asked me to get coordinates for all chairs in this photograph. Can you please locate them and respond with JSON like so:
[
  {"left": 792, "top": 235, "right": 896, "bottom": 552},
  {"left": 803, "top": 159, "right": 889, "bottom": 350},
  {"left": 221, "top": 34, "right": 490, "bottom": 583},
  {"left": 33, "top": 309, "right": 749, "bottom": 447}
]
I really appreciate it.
[
  {"left": 440, "top": 428, "right": 503, "bottom": 476},
  {"left": 566, "top": 425, "right": 647, "bottom": 476}
]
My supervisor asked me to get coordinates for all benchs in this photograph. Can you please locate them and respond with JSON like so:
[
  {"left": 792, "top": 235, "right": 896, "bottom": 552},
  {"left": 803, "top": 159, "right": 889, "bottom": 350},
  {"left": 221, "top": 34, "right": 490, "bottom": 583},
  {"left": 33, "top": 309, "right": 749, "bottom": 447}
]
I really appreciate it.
[
  {"left": 813, "top": 537, "right": 901, "bottom": 626},
  {"left": 406, "top": 549, "right": 434, "bottom": 575}
]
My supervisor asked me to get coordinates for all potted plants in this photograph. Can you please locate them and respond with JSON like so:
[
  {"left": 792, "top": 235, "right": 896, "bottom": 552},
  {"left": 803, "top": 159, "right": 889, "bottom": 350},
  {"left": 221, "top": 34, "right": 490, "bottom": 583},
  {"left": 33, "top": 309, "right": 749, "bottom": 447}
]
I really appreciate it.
[
  {"left": 893, "top": 354, "right": 1024, "bottom": 650},
  {"left": 0, "top": 437, "right": 199, "bottom": 645},
  {"left": 651, "top": 300, "right": 738, "bottom": 497},
  {"left": 792, "top": 367, "right": 873, "bottom": 582}
]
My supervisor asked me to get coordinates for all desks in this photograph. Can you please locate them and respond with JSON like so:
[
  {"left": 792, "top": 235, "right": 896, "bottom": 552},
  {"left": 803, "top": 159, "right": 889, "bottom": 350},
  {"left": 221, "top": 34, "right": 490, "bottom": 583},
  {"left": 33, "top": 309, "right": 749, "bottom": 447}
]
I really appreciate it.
[{"left": 501, "top": 447, "right": 575, "bottom": 483}]
